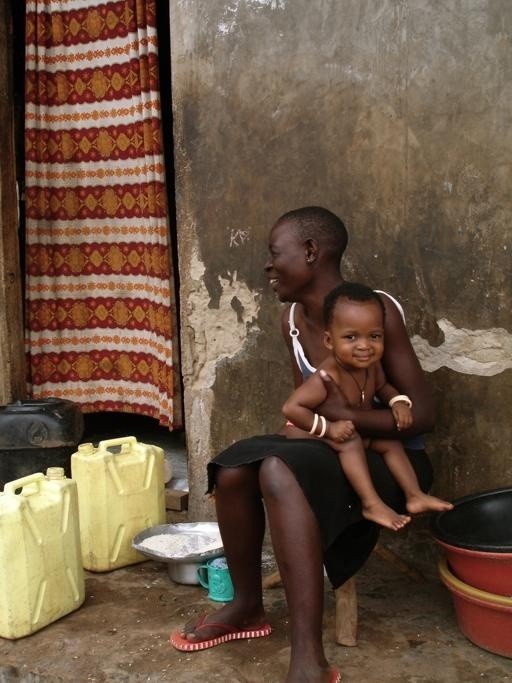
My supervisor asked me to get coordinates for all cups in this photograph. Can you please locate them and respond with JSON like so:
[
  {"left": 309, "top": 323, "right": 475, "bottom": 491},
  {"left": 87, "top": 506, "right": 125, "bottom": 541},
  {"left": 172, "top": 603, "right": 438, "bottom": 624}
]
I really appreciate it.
[{"left": 196, "top": 558, "right": 236, "bottom": 600}]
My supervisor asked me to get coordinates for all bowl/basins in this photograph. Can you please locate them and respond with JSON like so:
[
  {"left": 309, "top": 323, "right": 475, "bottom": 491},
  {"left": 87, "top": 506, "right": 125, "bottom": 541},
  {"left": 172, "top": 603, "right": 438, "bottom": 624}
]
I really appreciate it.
[
  {"left": 130, "top": 521, "right": 225, "bottom": 585},
  {"left": 436, "top": 488, "right": 512, "bottom": 661}
]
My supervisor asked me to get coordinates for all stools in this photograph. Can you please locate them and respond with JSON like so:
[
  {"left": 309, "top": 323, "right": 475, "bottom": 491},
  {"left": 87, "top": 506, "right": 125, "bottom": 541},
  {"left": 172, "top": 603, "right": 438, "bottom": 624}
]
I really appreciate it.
[{"left": 263, "top": 511, "right": 431, "bottom": 648}]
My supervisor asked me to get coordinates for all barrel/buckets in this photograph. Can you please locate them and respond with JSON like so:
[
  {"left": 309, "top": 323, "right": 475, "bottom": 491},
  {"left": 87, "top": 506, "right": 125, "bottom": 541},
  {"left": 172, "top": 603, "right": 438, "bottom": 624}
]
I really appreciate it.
[
  {"left": 1, "top": 394, "right": 83, "bottom": 490},
  {"left": 72, "top": 436, "right": 166, "bottom": 573},
  {"left": 0, "top": 465, "right": 85, "bottom": 642}
]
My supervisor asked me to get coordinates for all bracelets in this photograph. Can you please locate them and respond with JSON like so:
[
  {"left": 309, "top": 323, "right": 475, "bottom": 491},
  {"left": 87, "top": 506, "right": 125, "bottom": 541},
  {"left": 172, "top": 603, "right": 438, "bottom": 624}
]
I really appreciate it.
[
  {"left": 388, "top": 393, "right": 413, "bottom": 408},
  {"left": 316, "top": 415, "right": 327, "bottom": 437},
  {"left": 307, "top": 413, "right": 319, "bottom": 435}
]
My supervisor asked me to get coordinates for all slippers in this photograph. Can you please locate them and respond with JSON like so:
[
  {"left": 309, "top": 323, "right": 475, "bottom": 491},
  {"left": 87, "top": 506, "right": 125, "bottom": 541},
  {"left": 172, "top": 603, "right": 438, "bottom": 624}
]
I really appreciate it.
[
  {"left": 328, "top": 669, "right": 343, "bottom": 683},
  {"left": 169, "top": 610, "right": 272, "bottom": 652}
]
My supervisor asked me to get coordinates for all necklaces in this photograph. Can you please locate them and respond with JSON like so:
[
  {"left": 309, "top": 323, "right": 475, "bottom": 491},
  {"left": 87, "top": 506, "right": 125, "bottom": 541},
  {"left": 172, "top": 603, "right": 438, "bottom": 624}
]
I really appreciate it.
[{"left": 333, "top": 358, "right": 369, "bottom": 404}]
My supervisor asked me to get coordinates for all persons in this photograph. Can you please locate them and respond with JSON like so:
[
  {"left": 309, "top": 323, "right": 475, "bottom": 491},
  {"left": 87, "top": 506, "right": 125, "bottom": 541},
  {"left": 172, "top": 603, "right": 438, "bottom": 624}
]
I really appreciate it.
[
  {"left": 170, "top": 205, "right": 438, "bottom": 681},
  {"left": 280, "top": 281, "right": 454, "bottom": 534}
]
